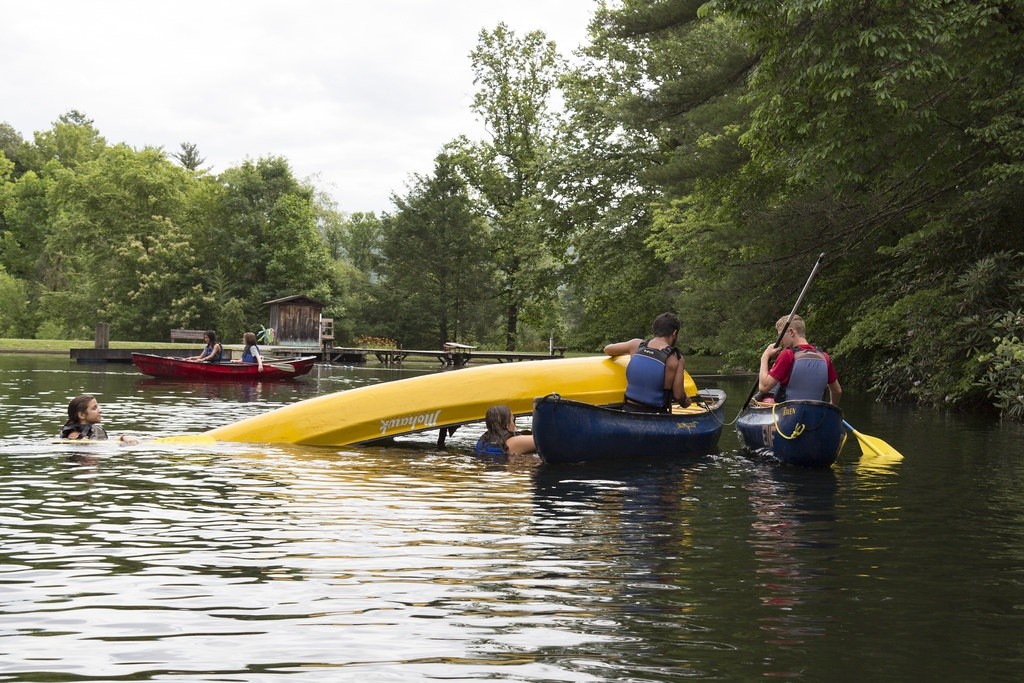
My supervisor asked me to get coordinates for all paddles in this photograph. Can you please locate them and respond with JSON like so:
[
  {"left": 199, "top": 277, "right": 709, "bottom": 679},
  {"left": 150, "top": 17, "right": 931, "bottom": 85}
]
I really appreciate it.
[
  {"left": 841, "top": 417, "right": 906, "bottom": 464},
  {"left": 234, "top": 361, "right": 296, "bottom": 373}
]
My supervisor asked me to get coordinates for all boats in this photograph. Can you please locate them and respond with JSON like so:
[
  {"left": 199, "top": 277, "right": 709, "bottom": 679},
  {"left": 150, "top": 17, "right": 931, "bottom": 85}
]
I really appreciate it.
[
  {"left": 130, "top": 352, "right": 317, "bottom": 381},
  {"left": 532, "top": 389, "right": 728, "bottom": 466},
  {"left": 734, "top": 383, "right": 845, "bottom": 470}
]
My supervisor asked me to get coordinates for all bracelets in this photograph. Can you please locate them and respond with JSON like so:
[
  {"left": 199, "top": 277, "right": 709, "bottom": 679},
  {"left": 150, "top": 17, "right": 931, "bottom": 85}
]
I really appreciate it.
[{"left": 120, "top": 435, "right": 125, "bottom": 442}]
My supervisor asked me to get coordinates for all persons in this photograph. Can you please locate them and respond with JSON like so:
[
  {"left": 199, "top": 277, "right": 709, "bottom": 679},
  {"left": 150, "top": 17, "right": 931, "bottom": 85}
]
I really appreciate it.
[
  {"left": 60, "top": 394, "right": 143, "bottom": 445},
  {"left": 758, "top": 314, "right": 843, "bottom": 406},
  {"left": 230, "top": 332, "right": 264, "bottom": 373},
  {"left": 474, "top": 407, "right": 535, "bottom": 458},
  {"left": 188, "top": 332, "right": 222, "bottom": 364},
  {"left": 604, "top": 312, "right": 691, "bottom": 412}
]
